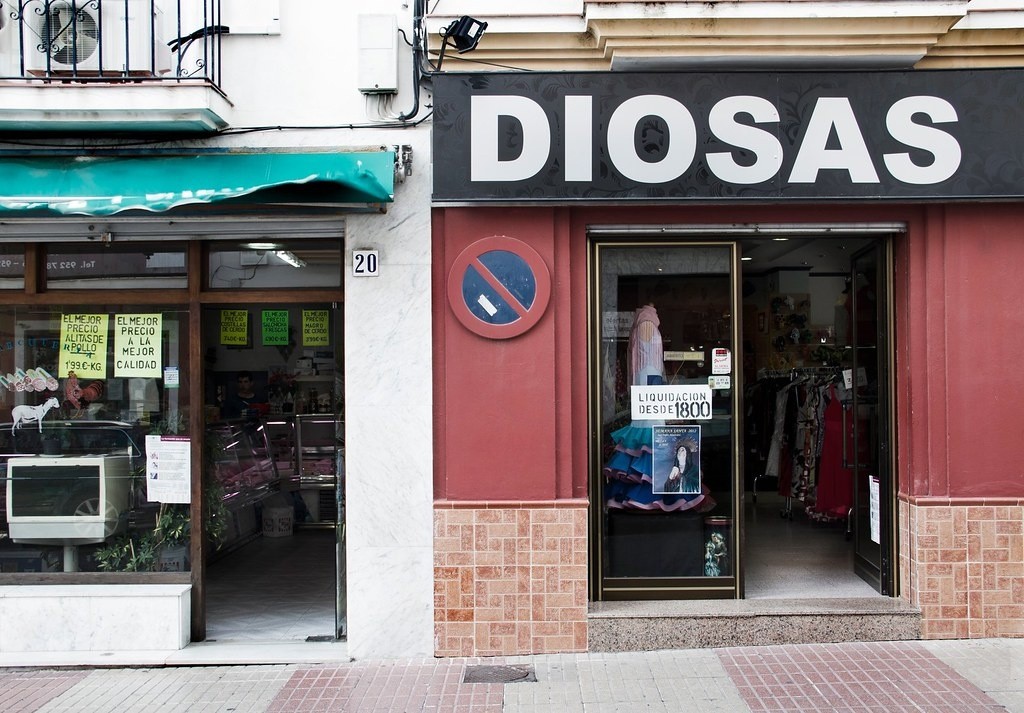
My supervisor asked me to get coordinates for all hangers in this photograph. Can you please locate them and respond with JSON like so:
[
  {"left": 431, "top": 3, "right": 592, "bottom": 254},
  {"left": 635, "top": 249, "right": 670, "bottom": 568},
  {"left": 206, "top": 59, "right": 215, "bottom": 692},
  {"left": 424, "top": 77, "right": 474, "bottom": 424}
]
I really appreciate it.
[{"left": 745, "top": 366, "right": 851, "bottom": 387}]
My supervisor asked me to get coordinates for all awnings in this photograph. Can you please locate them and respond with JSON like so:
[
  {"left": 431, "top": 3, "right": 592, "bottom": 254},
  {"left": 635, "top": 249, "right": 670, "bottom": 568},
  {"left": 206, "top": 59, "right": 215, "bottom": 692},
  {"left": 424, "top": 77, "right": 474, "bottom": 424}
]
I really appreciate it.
[{"left": 0, "top": 153, "right": 394, "bottom": 214}]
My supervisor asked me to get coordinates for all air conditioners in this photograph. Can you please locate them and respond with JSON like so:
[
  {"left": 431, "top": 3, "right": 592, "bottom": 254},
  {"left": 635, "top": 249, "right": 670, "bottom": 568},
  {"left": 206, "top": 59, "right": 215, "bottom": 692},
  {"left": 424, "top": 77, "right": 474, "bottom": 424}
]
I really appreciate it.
[{"left": 24, "top": 0, "right": 173, "bottom": 82}]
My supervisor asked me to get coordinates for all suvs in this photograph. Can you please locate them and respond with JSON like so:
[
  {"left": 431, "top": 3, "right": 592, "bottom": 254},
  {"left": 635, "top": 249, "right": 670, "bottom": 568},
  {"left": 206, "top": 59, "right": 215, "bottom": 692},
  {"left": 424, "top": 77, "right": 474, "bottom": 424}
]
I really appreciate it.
[{"left": 0, "top": 417, "right": 156, "bottom": 549}]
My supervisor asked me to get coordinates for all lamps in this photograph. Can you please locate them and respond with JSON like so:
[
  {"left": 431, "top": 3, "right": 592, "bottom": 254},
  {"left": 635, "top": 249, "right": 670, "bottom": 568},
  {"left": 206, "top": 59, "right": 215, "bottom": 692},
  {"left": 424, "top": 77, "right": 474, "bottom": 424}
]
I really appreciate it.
[{"left": 434, "top": 16, "right": 489, "bottom": 71}]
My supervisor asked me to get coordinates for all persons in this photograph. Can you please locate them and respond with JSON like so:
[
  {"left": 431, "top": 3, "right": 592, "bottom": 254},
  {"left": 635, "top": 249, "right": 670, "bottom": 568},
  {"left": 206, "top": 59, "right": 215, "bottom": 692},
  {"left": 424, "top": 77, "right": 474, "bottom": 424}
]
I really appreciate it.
[{"left": 228, "top": 373, "right": 267, "bottom": 409}]
